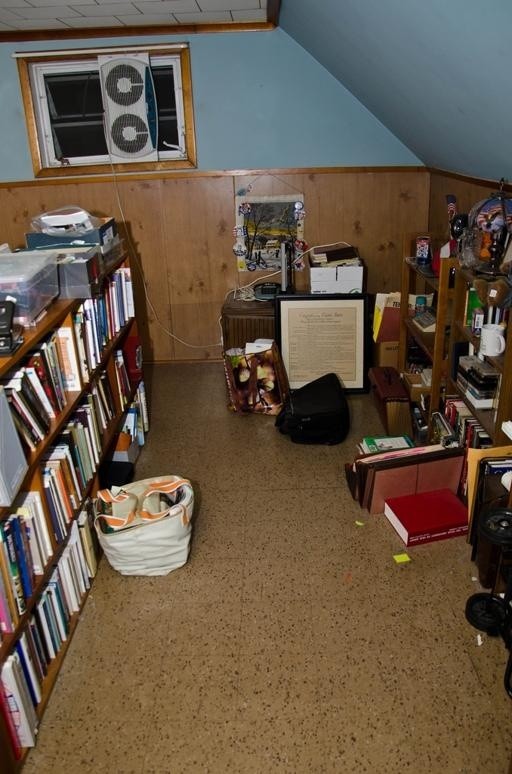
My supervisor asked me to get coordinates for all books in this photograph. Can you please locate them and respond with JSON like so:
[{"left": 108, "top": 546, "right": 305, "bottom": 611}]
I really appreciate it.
[
  {"left": 441, "top": 342, "right": 511, "bottom": 545},
  {"left": 383, "top": 489, "right": 469, "bottom": 548}
]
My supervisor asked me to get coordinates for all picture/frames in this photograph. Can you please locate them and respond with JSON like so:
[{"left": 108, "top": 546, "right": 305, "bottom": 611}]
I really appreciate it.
[
  {"left": 233, "top": 194, "right": 307, "bottom": 273},
  {"left": 273, "top": 292, "right": 372, "bottom": 396}
]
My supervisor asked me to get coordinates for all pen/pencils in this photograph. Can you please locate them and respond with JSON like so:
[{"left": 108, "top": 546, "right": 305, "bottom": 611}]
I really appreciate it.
[{"left": 411, "top": 363, "right": 423, "bottom": 374}]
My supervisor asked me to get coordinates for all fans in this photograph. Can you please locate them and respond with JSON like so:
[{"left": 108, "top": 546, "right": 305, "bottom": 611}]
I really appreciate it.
[{"left": 96, "top": 53, "right": 160, "bottom": 164}]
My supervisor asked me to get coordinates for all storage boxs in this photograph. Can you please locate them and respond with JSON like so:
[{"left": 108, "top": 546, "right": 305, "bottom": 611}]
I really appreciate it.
[
  {"left": 309, "top": 260, "right": 365, "bottom": 294},
  {"left": 0, "top": 216, "right": 114, "bottom": 327},
  {"left": 375, "top": 340, "right": 400, "bottom": 371}
]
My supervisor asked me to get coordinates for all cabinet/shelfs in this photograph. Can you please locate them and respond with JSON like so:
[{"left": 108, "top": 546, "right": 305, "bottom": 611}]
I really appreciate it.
[
  {"left": 0, "top": 246, "right": 150, "bottom": 774},
  {"left": 397, "top": 231, "right": 459, "bottom": 445},
  {"left": 443, "top": 264, "right": 512, "bottom": 515}
]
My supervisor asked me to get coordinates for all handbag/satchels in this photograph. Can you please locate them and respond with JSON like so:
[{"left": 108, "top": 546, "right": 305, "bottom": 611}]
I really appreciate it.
[
  {"left": 223, "top": 340, "right": 289, "bottom": 416},
  {"left": 94, "top": 474, "right": 194, "bottom": 577},
  {"left": 275, "top": 373, "right": 350, "bottom": 445}
]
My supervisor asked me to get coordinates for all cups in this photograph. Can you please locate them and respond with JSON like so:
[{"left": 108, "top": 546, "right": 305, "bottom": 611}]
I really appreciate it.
[{"left": 479, "top": 323, "right": 506, "bottom": 357}]
